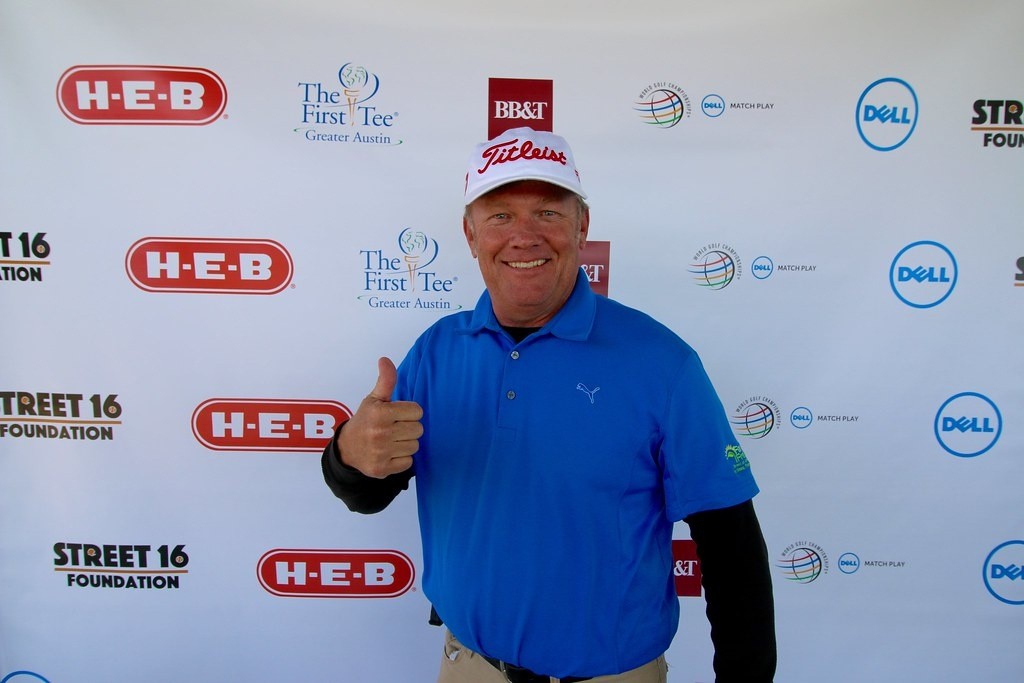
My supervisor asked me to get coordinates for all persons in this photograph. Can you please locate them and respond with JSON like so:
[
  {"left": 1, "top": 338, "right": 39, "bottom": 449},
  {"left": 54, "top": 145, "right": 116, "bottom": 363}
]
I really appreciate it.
[{"left": 320, "top": 125, "right": 778, "bottom": 683}]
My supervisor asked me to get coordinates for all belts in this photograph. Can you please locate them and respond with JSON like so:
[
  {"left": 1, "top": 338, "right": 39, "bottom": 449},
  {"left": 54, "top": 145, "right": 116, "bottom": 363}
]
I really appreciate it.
[{"left": 481, "top": 655, "right": 593, "bottom": 683}]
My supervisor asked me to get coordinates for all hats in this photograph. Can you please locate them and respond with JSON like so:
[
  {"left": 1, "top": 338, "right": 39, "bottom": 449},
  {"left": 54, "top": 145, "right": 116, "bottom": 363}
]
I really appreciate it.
[{"left": 464, "top": 126, "right": 588, "bottom": 206}]
